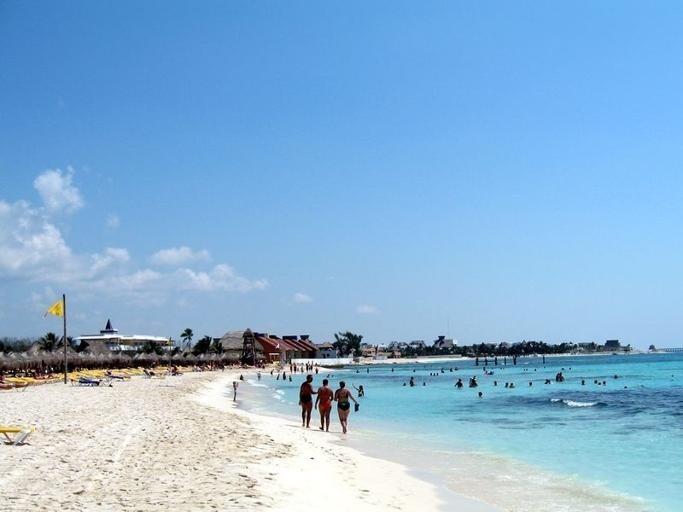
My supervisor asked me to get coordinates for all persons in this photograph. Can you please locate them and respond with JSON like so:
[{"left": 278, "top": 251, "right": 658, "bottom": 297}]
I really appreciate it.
[
  {"left": 105, "top": 361, "right": 225, "bottom": 387},
  {"left": 320, "top": 361, "right": 369, "bottom": 397},
  {"left": 225, "top": 361, "right": 318, "bottom": 401},
  {"left": 392, "top": 364, "right": 606, "bottom": 397},
  {"left": 297, "top": 373, "right": 318, "bottom": 428},
  {"left": 314, "top": 378, "right": 334, "bottom": 432},
  {"left": 333, "top": 380, "right": 358, "bottom": 434}
]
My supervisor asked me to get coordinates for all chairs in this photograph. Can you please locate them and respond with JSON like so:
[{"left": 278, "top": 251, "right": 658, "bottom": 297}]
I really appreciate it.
[{"left": 68, "top": 371, "right": 130, "bottom": 387}]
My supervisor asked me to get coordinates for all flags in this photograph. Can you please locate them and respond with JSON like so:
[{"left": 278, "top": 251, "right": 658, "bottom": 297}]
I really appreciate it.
[{"left": 42, "top": 299, "right": 64, "bottom": 319}]
